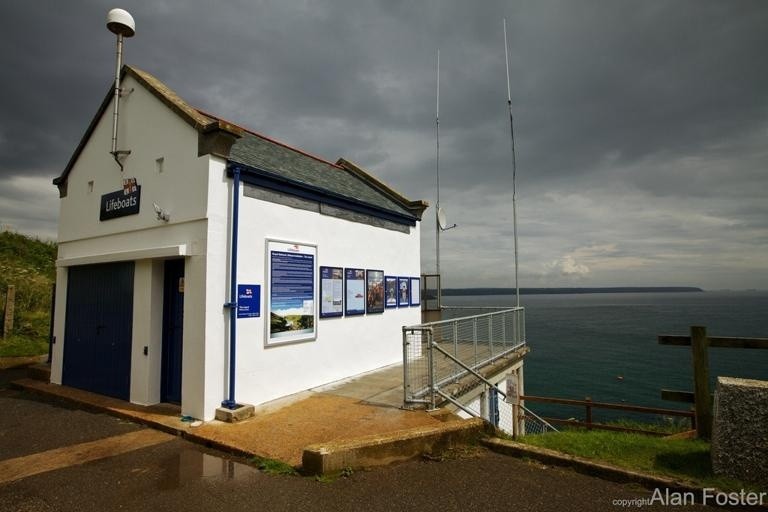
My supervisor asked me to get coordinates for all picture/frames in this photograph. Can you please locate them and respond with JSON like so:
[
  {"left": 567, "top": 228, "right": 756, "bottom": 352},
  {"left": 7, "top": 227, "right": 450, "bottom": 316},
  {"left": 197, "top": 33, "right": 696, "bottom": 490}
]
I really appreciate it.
[{"left": 263, "top": 238, "right": 421, "bottom": 348}]
